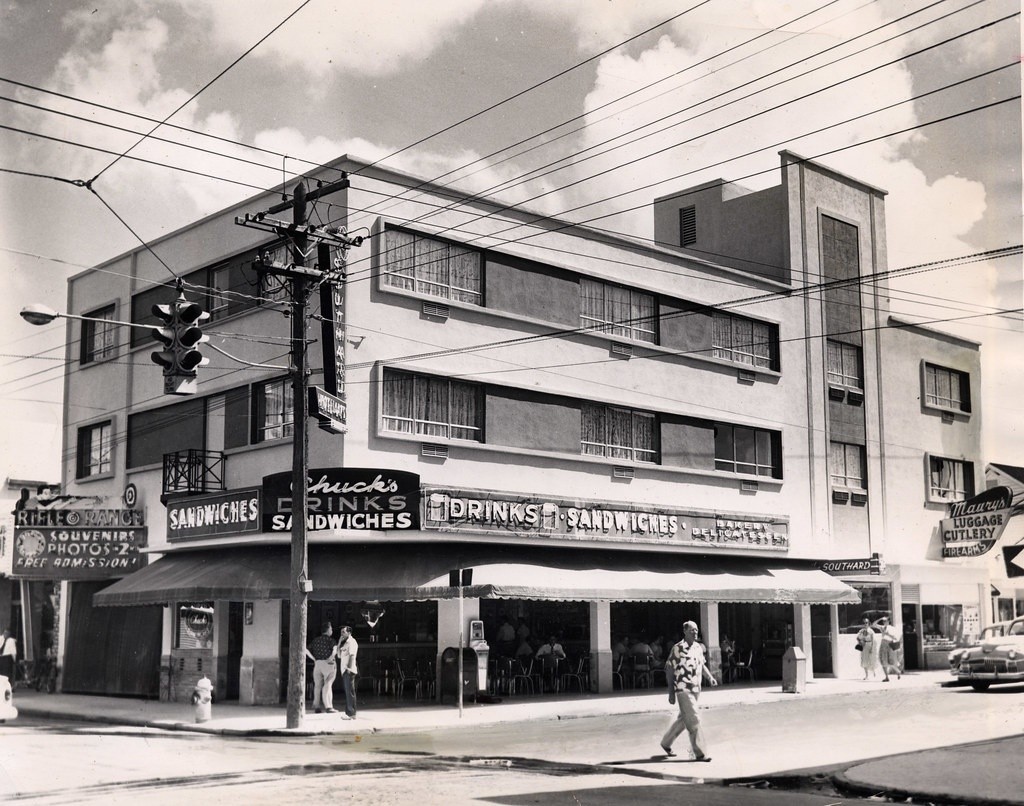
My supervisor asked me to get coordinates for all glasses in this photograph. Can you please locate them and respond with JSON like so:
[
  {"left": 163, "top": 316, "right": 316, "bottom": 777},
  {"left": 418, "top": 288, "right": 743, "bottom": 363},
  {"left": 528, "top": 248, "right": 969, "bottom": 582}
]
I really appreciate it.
[{"left": 863, "top": 622, "right": 869, "bottom": 624}]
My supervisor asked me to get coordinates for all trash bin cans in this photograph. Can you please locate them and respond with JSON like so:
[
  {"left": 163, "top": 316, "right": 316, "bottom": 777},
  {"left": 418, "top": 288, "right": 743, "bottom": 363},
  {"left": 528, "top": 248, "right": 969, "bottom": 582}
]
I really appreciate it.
[
  {"left": 780, "top": 646, "right": 807, "bottom": 694},
  {"left": 440, "top": 646, "right": 480, "bottom": 707}
]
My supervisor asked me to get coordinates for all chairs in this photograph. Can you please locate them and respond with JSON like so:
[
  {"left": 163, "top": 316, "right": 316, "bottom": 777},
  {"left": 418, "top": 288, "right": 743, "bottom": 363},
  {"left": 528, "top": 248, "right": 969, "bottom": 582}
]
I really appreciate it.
[{"left": 358, "top": 650, "right": 754, "bottom": 695}]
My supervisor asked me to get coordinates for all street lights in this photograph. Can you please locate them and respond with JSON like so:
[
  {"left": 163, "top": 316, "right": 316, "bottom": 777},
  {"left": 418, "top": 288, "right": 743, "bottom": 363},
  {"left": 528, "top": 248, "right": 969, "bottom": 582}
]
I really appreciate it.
[{"left": 20, "top": 302, "right": 314, "bottom": 729}]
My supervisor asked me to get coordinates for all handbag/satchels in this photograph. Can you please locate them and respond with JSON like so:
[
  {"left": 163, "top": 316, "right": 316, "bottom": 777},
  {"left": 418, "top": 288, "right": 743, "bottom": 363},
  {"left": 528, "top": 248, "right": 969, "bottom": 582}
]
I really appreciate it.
[
  {"left": 889, "top": 638, "right": 900, "bottom": 651},
  {"left": 855, "top": 641, "right": 863, "bottom": 651}
]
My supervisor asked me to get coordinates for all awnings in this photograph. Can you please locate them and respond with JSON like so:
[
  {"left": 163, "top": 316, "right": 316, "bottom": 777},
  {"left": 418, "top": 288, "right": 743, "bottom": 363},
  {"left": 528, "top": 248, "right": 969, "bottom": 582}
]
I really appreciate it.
[{"left": 92, "top": 542, "right": 863, "bottom": 606}]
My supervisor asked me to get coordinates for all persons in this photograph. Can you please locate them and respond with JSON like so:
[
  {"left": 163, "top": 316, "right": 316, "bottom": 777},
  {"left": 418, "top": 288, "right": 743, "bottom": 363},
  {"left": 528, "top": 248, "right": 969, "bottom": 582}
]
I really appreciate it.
[
  {"left": 612, "top": 632, "right": 678, "bottom": 685},
  {"left": 0, "top": 629, "right": 17, "bottom": 692},
  {"left": 306, "top": 622, "right": 340, "bottom": 713},
  {"left": 496, "top": 616, "right": 567, "bottom": 691},
  {"left": 856, "top": 618, "right": 877, "bottom": 680},
  {"left": 721, "top": 634, "right": 736, "bottom": 682},
  {"left": 46, "top": 640, "right": 52, "bottom": 658},
  {"left": 336, "top": 625, "right": 359, "bottom": 720},
  {"left": 872, "top": 616, "right": 901, "bottom": 682},
  {"left": 16, "top": 488, "right": 29, "bottom": 510},
  {"left": 660, "top": 620, "right": 718, "bottom": 763}
]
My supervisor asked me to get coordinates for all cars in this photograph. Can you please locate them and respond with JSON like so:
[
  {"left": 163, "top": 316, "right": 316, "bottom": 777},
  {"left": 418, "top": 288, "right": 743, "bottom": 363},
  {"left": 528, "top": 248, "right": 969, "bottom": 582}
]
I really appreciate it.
[
  {"left": 958, "top": 615, "right": 1024, "bottom": 691},
  {"left": 949, "top": 620, "right": 1017, "bottom": 684}
]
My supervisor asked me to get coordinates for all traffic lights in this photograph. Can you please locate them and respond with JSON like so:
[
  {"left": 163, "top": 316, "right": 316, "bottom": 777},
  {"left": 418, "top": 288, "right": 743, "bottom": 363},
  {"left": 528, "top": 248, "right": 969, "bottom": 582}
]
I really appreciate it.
[
  {"left": 195, "top": 308, "right": 210, "bottom": 368},
  {"left": 152, "top": 303, "right": 175, "bottom": 375},
  {"left": 175, "top": 301, "right": 202, "bottom": 374}
]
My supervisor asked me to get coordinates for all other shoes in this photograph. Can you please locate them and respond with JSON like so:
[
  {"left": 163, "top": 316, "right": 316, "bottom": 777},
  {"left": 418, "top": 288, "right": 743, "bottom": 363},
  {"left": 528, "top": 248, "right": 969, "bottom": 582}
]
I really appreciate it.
[
  {"left": 341, "top": 713, "right": 356, "bottom": 720},
  {"left": 326, "top": 707, "right": 339, "bottom": 713},
  {"left": 898, "top": 671, "right": 901, "bottom": 680},
  {"left": 660, "top": 744, "right": 677, "bottom": 756},
  {"left": 314, "top": 708, "right": 321, "bottom": 713},
  {"left": 882, "top": 678, "right": 889, "bottom": 682},
  {"left": 696, "top": 754, "right": 712, "bottom": 762}
]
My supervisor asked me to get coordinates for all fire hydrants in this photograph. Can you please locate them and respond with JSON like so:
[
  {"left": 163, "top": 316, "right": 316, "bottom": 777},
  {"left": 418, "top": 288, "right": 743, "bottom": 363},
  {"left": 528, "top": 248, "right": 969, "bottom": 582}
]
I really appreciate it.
[{"left": 190, "top": 675, "right": 213, "bottom": 723}]
